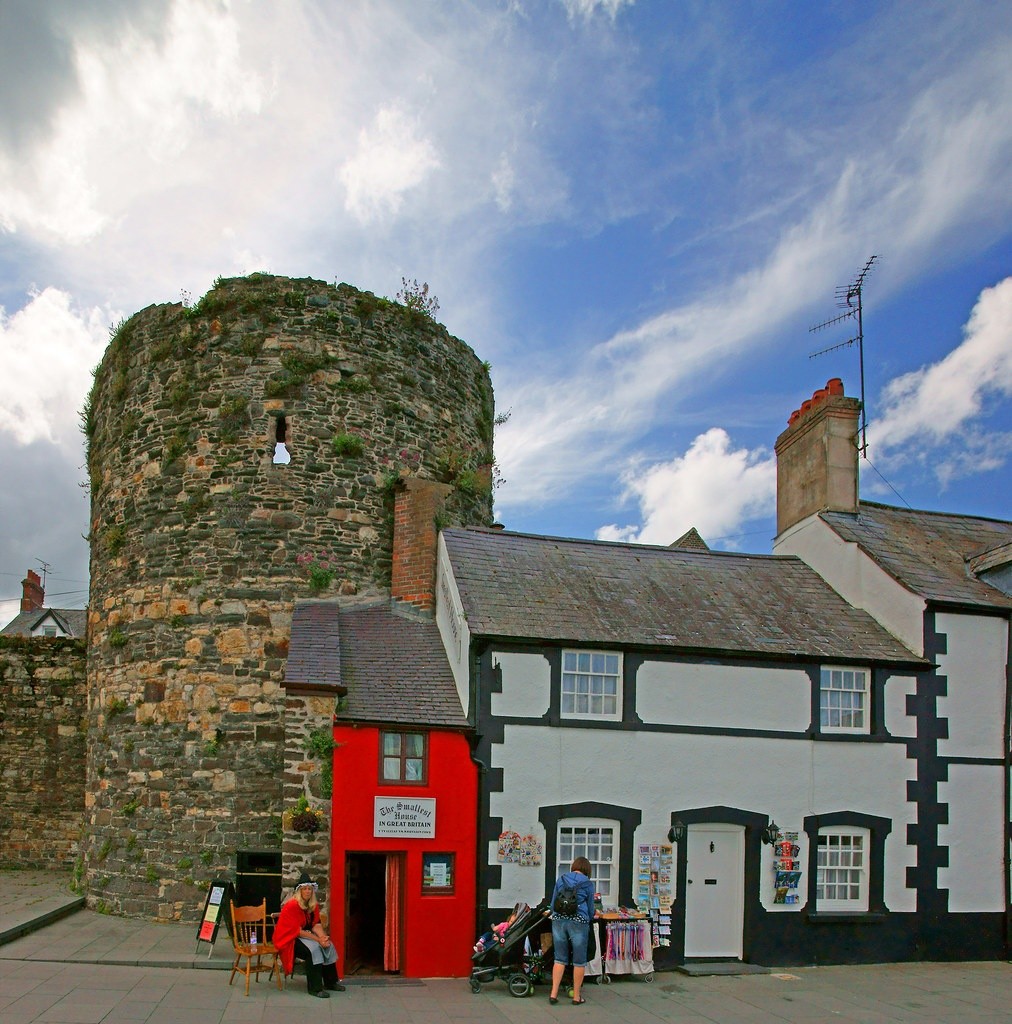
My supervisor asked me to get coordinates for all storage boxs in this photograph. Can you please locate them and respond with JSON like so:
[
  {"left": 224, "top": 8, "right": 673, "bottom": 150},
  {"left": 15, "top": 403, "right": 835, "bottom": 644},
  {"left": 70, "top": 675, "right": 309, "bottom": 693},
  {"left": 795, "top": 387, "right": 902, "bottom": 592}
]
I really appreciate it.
[{"left": 540, "top": 933, "right": 552, "bottom": 954}]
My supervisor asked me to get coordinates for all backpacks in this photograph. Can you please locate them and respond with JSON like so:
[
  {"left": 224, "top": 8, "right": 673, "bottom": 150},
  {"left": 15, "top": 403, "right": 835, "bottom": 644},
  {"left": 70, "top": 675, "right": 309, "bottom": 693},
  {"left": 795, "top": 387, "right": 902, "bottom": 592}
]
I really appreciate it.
[{"left": 554, "top": 876, "right": 590, "bottom": 915}]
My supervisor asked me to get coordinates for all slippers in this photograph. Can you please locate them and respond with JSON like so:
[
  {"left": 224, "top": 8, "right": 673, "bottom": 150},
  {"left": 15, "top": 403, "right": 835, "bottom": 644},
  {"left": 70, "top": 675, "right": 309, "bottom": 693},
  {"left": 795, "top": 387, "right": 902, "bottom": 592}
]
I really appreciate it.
[
  {"left": 549, "top": 995, "right": 558, "bottom": 1005},
  {"left": 572, "top": 995, "right": 586, "bottom": 1005}
]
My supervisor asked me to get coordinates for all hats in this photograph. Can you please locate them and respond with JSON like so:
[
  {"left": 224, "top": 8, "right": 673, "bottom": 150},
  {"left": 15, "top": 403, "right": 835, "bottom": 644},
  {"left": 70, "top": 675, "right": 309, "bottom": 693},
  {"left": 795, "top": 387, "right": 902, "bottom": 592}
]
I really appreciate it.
[{"left": 294, "top": 873, "right": 319, "bottom": 893}]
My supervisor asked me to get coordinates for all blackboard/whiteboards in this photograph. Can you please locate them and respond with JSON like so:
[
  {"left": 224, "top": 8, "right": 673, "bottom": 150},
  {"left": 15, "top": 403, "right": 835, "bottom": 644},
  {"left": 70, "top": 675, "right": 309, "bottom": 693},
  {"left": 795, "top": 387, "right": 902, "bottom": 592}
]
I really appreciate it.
[{"left": 195, "top": 880, "right": 231, "bottom": 944}]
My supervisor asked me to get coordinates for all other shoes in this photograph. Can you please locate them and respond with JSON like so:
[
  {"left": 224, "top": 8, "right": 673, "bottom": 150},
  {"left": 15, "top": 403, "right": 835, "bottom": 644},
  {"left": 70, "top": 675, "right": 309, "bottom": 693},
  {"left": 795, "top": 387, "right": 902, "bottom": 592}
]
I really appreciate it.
[
  {"left": 323, "top": 984, "right": 345, "bottom": 991},
  {"left": 476, "top": 942, "right": 483, "bottom": 947},
  {"left": 308, "top": 990, "right": 330, "bottom": 998},
  {"left": 473, "top": 946, "right": 479, "bottom": 953}
]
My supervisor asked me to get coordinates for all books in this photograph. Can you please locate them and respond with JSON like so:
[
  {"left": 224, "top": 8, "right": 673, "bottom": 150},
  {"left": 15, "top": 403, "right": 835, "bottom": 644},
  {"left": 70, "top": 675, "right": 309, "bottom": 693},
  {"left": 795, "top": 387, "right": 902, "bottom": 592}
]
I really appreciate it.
[
  {"left": 637, "top": 843, "right": 676, "bottom": 949},
  {"left": 772, "top": 831, "right": 803, "bottom": 904}
]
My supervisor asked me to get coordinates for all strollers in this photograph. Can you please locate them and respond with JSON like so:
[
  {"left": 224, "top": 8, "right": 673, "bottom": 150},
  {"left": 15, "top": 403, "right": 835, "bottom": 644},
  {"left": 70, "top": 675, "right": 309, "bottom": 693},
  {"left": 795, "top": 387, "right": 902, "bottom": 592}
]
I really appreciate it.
[{"left": 469, "top": 897, "right": 554, "bottom": 999}]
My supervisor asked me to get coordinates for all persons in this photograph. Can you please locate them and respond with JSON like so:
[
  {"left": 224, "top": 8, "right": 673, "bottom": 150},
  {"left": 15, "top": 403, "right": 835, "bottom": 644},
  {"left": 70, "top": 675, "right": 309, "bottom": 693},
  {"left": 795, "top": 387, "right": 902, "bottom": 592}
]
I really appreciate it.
[
  {"left": 472, "top": 914, "right": 519, "bottom": 953},
  {"left": 271, "top": 871, "right": 347, "bottom": 999},
  {"left": 543, "top": 856, "right": 596, "bottom": 1006}
]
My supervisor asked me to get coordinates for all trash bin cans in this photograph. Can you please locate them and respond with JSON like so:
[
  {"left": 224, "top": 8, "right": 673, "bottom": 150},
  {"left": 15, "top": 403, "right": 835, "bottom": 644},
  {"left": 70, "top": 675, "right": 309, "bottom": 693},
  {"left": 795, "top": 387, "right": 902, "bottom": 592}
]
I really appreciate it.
[{"left": 232, "top": 851, "right": 283, "bottom": 944}]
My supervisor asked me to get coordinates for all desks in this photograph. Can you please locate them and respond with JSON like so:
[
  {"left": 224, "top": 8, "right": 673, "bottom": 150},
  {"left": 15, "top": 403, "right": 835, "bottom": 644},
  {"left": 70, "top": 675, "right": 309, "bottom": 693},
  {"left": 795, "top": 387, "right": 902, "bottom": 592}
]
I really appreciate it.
[{"left": 542, "top": 913, "right": 654, "bottom": 985}]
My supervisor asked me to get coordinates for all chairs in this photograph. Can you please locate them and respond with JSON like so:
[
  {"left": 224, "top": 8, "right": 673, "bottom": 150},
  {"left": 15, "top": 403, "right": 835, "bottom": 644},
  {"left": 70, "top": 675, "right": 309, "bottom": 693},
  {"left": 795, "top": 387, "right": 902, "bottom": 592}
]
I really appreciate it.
[{"left": 229, "top": 897, "right": 305, "bottom": 996}]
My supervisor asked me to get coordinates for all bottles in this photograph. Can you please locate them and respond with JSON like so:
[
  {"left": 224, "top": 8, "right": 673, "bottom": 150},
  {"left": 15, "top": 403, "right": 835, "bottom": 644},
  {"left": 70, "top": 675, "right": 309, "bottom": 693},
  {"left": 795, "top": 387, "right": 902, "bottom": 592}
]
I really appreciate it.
[{"left": 250, "top": 932, "right": 257, "bottom": 953}]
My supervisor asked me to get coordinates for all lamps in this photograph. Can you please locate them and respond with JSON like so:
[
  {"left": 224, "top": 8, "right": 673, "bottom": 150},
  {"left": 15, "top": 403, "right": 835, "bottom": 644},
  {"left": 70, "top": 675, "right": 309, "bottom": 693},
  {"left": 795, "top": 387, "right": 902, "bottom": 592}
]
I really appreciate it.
[
  {"left": 668, "top": 818, "right": 686, "bottom": 843},
  {"left": 762, "top": 820, "right": 780, "bottom": 847}
]
